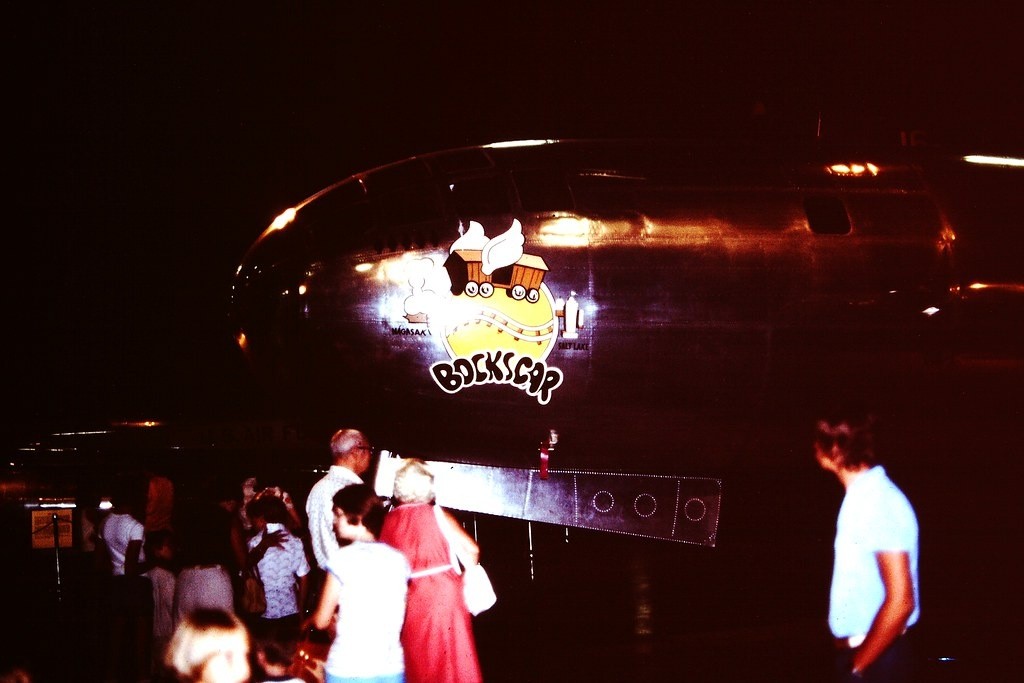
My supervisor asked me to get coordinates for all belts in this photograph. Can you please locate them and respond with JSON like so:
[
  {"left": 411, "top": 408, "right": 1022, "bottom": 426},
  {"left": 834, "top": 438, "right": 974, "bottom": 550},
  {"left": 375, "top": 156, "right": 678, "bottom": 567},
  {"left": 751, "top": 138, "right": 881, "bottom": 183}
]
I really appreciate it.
[{"left": 182, "top": 563, "right": 218, "bottom": 571}]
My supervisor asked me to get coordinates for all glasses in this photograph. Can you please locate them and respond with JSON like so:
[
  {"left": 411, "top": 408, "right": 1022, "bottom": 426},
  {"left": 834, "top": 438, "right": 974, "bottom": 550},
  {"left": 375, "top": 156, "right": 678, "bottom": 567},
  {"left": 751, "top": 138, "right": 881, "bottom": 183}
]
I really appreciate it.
[{"left": 358, "top": 446, "right": 375, "bottom": 455}]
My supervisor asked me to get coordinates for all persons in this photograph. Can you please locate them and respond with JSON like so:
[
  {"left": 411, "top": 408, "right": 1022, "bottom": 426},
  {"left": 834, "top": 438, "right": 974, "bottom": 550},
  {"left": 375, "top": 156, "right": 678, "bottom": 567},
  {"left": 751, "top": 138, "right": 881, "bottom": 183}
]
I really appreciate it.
[
  {"left": 305, "top": 429, "right": 372, "bottom": 571},
  {"left": 812, "top": 406, "right": 920, "bottom": 683},
  {"left": 83, "top": 469, "right": 311, "bottom": 683},
  {"left": 379, "top": 456, "right": 485, "bottom": 683},
  {"left": 312, "top": 482, "right": 412, "bottom": 683}
]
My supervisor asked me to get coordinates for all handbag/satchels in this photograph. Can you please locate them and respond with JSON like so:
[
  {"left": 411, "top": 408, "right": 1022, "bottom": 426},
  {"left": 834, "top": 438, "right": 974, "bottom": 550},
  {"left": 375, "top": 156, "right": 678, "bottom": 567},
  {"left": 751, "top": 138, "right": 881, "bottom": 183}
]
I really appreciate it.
[{"left": 461, "top": 564, "right": 497, "bottom": 616}]
